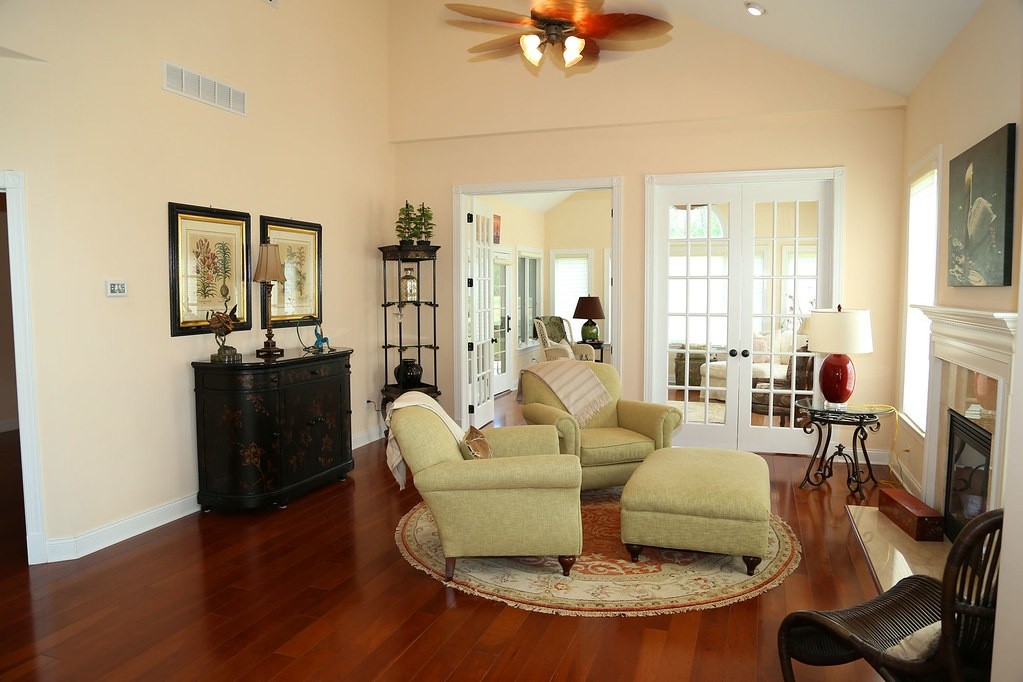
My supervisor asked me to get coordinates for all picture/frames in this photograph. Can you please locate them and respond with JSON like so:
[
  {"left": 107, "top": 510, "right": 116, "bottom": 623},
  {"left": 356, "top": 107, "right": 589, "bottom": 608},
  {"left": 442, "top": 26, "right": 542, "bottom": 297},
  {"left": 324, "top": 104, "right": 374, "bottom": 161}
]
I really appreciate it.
[
  {"left": 167, "top": 201, "right": 252, "bottom": 338},
  {"left": 493, "top": 214, "right": 502, "bottom": 244},
  {"left": 260, "top": 215, "right": 323, "bottom": 330},
  {"left": 948, "top": 123, "right": 1016, "bottom": 288}
]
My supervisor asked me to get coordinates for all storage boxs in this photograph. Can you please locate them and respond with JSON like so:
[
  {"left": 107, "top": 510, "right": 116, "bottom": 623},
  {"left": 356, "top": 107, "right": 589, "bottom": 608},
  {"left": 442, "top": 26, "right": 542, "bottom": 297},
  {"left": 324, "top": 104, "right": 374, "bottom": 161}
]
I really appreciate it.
[{"left": 876, "top": 488, "right": 945, "bottom": 544}]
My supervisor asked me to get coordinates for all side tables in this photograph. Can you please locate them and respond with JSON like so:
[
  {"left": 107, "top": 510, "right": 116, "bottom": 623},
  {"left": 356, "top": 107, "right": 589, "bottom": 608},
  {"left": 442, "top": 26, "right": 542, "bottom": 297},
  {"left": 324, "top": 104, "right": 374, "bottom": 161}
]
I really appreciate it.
[{"left": 376, "top": 245, "right": 442, "bottom": 448}]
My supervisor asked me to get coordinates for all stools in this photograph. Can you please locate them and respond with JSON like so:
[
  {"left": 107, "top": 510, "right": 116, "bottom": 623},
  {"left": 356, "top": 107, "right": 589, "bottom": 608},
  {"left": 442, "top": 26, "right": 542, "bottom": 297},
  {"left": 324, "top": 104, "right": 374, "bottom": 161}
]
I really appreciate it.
[
  {"left": 620, "top": 447, "right": 772, "bottom": 577},
  {"left": 674, "top": 343, "right": 718, "bottom": 387}
]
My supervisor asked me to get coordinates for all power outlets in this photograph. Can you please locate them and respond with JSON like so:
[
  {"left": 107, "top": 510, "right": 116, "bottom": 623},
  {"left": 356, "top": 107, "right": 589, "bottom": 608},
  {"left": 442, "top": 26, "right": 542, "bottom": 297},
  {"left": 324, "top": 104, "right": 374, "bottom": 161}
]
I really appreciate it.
[
  {"left": 364, "top": 396, "right": 371, "bottom": 408},
  {"left": 906, "top": 444, "right": 912, "bottom": 459}
]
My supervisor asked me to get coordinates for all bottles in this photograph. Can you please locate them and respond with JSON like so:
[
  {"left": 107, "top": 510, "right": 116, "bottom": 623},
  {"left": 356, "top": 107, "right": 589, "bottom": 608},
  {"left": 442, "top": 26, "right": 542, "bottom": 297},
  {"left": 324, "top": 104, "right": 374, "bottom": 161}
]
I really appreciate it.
[
  {"left": 400, "top": 268, "right": 418, "bottom": 301},
  {"left": 394, "top": 358, "right": 423, "bottom": 389}
]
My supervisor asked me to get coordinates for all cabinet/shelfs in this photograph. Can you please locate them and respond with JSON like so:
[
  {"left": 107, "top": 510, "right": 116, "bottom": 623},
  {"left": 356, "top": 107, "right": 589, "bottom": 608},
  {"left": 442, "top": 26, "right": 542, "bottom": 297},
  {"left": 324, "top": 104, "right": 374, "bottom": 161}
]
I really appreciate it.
[{"left": 190, "top": 346, "right": 356, "bottom": 519}]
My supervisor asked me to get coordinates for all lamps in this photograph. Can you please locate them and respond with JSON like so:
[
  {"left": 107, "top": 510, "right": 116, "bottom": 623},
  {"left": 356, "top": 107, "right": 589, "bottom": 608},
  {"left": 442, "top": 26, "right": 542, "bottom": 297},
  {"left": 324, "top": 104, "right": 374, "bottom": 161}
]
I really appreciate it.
[
  {"left": 573, "top": 294, "right": 605, "bottom": 342},
  {"left": 252, "top": 237, "right": 288, "bottom": 357},
  {"left": 975, "top": 373, "right": 997, "bottom": 418},
  {"left": 806, "top": 302, "right": 874, "bottom": 404},
  {"left": 797, "top": 317, "right": 809, "bottom": 351},
  {"left": 518, "top": 24, "right": 586, "bottom": 68}
]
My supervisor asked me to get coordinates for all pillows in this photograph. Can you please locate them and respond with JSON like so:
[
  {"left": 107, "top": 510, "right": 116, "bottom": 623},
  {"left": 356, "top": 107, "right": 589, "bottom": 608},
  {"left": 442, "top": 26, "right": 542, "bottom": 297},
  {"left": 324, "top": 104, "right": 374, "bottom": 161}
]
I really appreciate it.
[
  {"left": 780, "top": 330, "right": 793, "bottom": 366},
  {"left": 751, "top": 333, "right": 771, "bottom": 363},
  {"left": 882, "top": 614, "right": 958, "bottom": 663},
  {"left": 459, "top": 424, "right": 494, "bottom": 461}
]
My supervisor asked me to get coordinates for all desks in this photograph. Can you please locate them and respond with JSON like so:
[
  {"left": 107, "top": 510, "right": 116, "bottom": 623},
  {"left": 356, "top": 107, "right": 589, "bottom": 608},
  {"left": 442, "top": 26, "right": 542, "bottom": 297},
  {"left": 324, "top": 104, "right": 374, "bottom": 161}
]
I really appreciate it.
[
  {"left": 577, "top": 341, "right": 604, "bottom": 363},
  {"left": 795, "top": 398, "right": 895, "bottom": 501}
]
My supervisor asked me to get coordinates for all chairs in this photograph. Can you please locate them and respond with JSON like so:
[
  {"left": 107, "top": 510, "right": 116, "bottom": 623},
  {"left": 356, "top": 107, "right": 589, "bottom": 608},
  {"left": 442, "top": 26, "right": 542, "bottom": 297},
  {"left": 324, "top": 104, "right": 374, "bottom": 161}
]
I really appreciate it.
[
  {"left": 389, "top": 391, "right": 584, "bottom": 583},
  {"left": 534, "top": 317, "right": 596, "bottom": 362},
  {"left": 751, "top": 344, "right": 815, "bottom": 428},
  {"left": 520, "top": 360, "right": 683, "bottom": 491},
  {"left": 775, "top": 507, "right": 1004, "bottom": 682}
]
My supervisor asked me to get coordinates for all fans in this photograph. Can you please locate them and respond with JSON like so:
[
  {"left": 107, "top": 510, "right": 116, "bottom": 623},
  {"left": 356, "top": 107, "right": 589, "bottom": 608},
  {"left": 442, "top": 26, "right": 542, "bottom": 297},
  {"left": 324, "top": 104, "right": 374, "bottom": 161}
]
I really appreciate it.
[{"left": 443, "top": 0, "right": 675, "bottom": 56}]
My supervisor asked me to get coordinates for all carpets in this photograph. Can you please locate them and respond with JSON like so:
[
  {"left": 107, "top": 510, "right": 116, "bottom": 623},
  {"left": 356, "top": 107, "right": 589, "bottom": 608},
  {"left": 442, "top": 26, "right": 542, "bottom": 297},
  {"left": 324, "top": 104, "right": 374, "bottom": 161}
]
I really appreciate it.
[
  {"left": 667, "top": 400, "right": 726, "bottom": 424},
  {"left": 394, "top": 485, "right": 803, "bottom": 619}
]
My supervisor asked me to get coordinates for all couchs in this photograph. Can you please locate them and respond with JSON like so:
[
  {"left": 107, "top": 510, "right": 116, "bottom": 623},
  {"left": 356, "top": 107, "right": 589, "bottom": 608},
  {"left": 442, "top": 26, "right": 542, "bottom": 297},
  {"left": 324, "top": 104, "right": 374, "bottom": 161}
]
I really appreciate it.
[{"left": 699, "top": 329, "right": 809, "bottom": 401}]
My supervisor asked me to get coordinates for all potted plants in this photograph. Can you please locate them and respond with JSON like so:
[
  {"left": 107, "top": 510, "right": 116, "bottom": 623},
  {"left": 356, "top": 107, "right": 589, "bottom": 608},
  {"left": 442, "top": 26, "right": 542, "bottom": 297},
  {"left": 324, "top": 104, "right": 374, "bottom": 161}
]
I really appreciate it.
[
  {"left": 414, "top": 201, "right": 436, "bottom": 246},
  {"left": 395, "top": 200, "right": 417, "bottom": 245}
]
